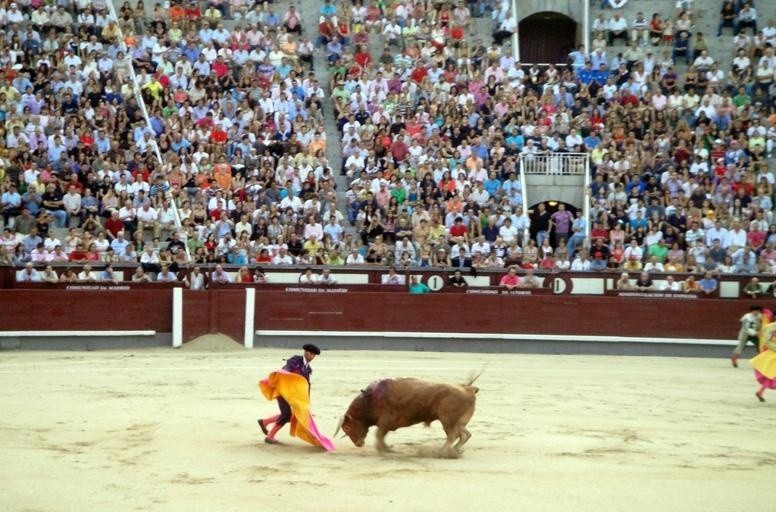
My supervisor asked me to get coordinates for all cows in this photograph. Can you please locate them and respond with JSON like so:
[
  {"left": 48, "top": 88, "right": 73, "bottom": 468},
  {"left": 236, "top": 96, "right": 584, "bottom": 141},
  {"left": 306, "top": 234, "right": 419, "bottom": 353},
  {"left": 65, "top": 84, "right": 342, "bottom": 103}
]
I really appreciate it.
[{"left": 333, "top": 374, "right": 480, "bottom": 458}]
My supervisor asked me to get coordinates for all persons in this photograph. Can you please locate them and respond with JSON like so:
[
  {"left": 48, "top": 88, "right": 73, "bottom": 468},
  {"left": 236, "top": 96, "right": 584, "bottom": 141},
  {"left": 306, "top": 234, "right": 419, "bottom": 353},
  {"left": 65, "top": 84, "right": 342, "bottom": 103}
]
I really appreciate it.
[
  {"left": 730, "top": 305, "right": 764, "bottom": 368},
  {"left": 258, "top": 344, "right": 320, "bottom": 444},
  {"left": 749, "top": 320, "right": 776, "bottom": 402}
]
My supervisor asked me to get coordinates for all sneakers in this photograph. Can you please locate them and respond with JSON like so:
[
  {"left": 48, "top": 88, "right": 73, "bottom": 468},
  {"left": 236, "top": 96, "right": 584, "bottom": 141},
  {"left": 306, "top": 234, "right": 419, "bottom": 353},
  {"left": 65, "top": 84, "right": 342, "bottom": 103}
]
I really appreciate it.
[{"left": 259, "top": 419, "right": 277, "bottom": 442}]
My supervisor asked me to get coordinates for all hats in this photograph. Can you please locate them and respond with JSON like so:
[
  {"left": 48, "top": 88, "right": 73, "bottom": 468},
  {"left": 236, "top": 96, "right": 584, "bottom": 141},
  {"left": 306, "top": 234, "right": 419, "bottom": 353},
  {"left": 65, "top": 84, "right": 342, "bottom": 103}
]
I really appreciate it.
[{"left": 303, "top": 344, "right": 320, "bottom": 355}]
